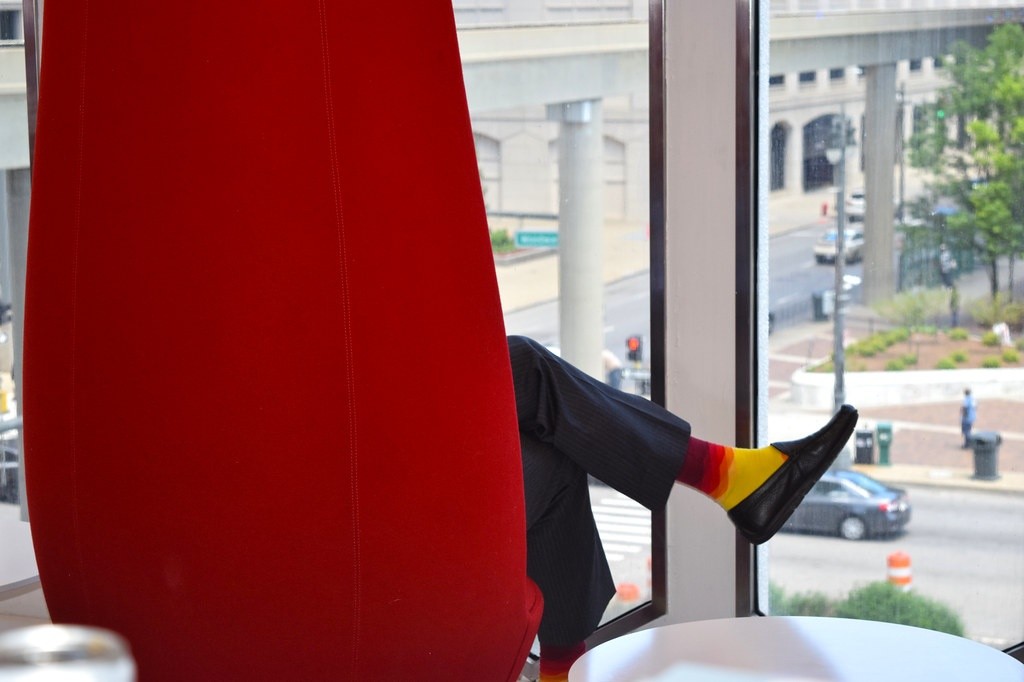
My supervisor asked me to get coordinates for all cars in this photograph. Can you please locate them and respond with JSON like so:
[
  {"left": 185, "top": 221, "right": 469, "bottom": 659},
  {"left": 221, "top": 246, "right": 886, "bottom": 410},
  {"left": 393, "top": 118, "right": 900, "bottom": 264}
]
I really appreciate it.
[
  {"left": 814, "top": 229, "right": 865, "bottom": 266},
  {"left": 778, "top": 469, "right": 909, "bottom": 538}
]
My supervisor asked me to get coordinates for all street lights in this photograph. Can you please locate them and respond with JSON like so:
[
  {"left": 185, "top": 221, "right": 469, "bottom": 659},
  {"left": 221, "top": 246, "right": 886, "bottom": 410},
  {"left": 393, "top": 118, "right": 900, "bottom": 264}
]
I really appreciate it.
[{"left": 825, "top": 119, "right": 845, "bottom": 420}]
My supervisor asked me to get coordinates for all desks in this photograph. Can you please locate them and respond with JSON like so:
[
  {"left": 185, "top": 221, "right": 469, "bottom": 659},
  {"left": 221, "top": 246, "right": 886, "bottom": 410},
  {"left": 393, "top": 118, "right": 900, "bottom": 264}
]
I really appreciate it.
[
  {"left": 567, "top": 615, "right": 1024, "bottom": 682},
  {"left": 1, "top": 518, "right": 41, "bottom": 602}
]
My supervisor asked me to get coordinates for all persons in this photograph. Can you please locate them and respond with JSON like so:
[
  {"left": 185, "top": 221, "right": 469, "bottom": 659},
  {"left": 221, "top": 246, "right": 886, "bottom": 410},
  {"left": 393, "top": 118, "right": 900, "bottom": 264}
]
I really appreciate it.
[
  {"left": 961, "top": 391, "right": 975, "bottom": 450},
  {"left": 506, "top": 332, "right": 860, "bottom": 682}
]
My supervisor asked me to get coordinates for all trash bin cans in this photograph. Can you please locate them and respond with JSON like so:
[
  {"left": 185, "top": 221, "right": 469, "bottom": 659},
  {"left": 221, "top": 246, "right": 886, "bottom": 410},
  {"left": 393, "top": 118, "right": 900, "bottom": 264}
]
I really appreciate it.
[
  {"left": 972, "top": 430, "right": 1004, "bottom": 480},
  {"left": 855, "top": 431, "right": 875, "bottom": 465}
]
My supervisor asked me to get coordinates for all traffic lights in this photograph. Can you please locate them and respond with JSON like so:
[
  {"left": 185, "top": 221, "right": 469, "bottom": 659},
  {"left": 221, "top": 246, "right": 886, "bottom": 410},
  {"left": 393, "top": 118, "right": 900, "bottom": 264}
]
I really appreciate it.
[
  {"left": 935, "top": 97, "right": 945, "bottom": 130},
  {"left": 628, "top": 336, "right": 642, "bottom": 362}
]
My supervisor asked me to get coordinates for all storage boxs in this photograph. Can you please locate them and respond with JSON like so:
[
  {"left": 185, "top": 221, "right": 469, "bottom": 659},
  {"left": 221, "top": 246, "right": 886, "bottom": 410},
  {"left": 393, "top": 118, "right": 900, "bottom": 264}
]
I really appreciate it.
[
  {"left": 970, "top": 434, "right": 1002, "bottom": 481},
  {"left": 855, "top": 429, "right": 873, "bottom": 462},
  {"left": 877, "top": 425, "right": 893, "bottom": 463}
]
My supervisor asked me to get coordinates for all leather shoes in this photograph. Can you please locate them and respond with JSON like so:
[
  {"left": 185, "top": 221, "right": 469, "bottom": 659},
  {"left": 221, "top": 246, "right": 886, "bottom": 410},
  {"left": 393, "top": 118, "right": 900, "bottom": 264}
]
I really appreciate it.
[{"left": 727, "top": 405, "right": 859, "bottom": 544}]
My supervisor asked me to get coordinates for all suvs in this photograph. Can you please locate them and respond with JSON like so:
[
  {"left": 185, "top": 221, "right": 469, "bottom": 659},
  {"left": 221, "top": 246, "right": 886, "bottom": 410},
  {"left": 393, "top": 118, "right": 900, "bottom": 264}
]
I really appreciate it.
[{"left": 844, "top": 191, "right": 902, "bottom": 223}]
